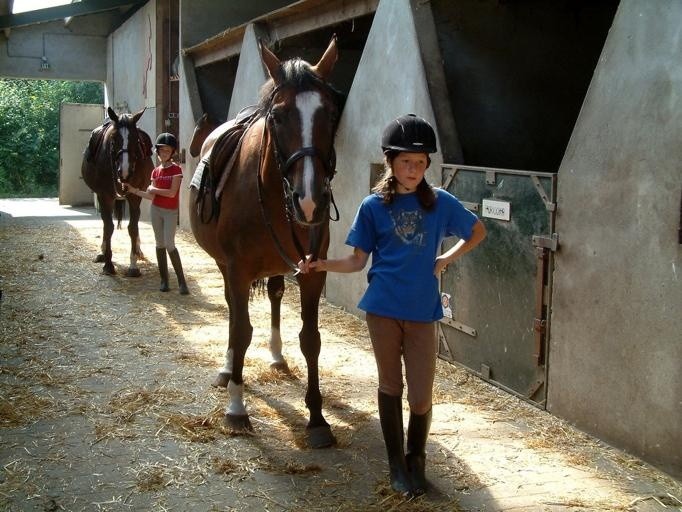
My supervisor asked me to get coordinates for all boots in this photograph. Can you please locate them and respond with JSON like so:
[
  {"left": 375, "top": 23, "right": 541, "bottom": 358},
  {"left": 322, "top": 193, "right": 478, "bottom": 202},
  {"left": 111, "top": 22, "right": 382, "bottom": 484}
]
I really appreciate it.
[
  {"left": 156, "top": 247, "right": 169, "bottom": 291},
  {"left": 378, "top": 388, "right": 432, "bottom": 491},
  {"left": 171, "top": 251, "right": 189, "bottom": 294}
]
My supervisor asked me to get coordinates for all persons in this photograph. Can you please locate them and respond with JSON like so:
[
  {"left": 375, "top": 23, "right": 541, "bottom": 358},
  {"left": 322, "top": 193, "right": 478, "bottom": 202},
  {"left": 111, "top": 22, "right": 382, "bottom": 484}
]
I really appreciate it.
[
  {"left": 119, "top": 133, "right": 189, "bottom": 295},
  {"left": 296, "top": 112, "right": 487, "bottom": 498}
]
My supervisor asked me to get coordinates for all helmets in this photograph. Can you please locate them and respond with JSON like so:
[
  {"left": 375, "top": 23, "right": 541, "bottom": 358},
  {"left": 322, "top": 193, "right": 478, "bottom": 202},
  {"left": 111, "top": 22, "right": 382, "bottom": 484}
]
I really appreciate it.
[
  {"left": 154, "top": 133, "right": 177, "bottom": 148},
  {"left": 381, "top": 114, "right": 436, "bottom": 153}
]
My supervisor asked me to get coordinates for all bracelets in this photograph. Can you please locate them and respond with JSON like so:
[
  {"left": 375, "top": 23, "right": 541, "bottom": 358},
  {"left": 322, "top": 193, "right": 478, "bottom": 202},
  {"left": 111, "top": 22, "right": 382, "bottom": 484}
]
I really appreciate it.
[{"left": 134, "top": 188, "right": 140, "bottom": 195}]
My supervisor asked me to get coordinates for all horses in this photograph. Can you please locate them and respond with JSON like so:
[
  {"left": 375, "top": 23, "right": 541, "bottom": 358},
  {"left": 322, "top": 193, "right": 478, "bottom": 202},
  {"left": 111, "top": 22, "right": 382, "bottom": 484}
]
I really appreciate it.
[
  {"left": 82, "top": 106, "right": 154, "bottom": 277},
  {"left": 190, "top": 111, "right": 223, "bottom": 157},
  {"left": 190, "top": 32, "right": 341, "bottom": 451}
]
